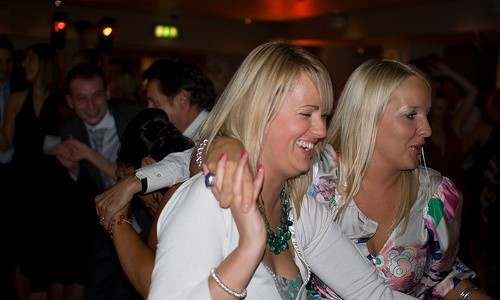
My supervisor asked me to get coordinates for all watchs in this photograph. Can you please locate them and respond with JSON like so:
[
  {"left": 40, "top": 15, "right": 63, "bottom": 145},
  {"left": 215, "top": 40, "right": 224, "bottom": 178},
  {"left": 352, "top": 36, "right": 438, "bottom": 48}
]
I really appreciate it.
[{"left": 135, "top": 171, "right": 147, "bottom": 194}]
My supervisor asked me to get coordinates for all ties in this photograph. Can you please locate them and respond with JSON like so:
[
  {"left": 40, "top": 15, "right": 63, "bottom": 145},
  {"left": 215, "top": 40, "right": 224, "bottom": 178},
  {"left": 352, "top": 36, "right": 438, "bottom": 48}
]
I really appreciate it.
[{"left": 90, "top": 128, "right": 112, "bottom": 190}]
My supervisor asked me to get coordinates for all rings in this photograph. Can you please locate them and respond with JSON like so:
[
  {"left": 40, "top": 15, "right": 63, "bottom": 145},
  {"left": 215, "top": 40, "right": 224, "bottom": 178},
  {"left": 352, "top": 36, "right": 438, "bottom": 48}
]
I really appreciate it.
[
  {"left": 100, "top": 218, "right": 104, "bottom": 220},
  {"left": 205, "top": 174, "right": 215, "bottom": 187}
]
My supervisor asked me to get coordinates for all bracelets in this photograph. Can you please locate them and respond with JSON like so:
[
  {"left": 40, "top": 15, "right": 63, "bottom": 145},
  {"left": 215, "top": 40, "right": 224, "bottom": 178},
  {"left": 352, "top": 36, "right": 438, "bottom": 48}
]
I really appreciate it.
[
  {"left": 211, "top": 269, "right": 247, "bottom": 298},
  {"left": 196, "top": 137, "right": 208, "bottom": 171},
  {"left": 459, "top": 287, "right": 478, "bottom": 300},
  {"left": 107, "top": 215, "right": 132, "bottom": 238}
]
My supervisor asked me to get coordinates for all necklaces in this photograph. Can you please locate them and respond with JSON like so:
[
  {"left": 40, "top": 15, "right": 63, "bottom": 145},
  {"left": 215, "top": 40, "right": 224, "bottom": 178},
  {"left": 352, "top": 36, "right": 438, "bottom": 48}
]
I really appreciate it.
[{"left": 256, "top": 186, "right": 294, "bottom": 255}]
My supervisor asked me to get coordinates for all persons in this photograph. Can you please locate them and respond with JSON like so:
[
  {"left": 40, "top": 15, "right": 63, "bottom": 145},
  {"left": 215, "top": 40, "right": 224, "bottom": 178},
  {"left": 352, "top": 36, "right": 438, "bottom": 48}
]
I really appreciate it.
[
  {"left": 189, "top": 57, "right": 489, "bottom": 300},
  {"left": 428, "top": 68, "right": 500, "bottom": 300},
  {"left": 0, "top": 39, "right": 231, "bottom": 300},
  {"left": 148, "top": 41, "right": 419, "bottom": 300}
]
